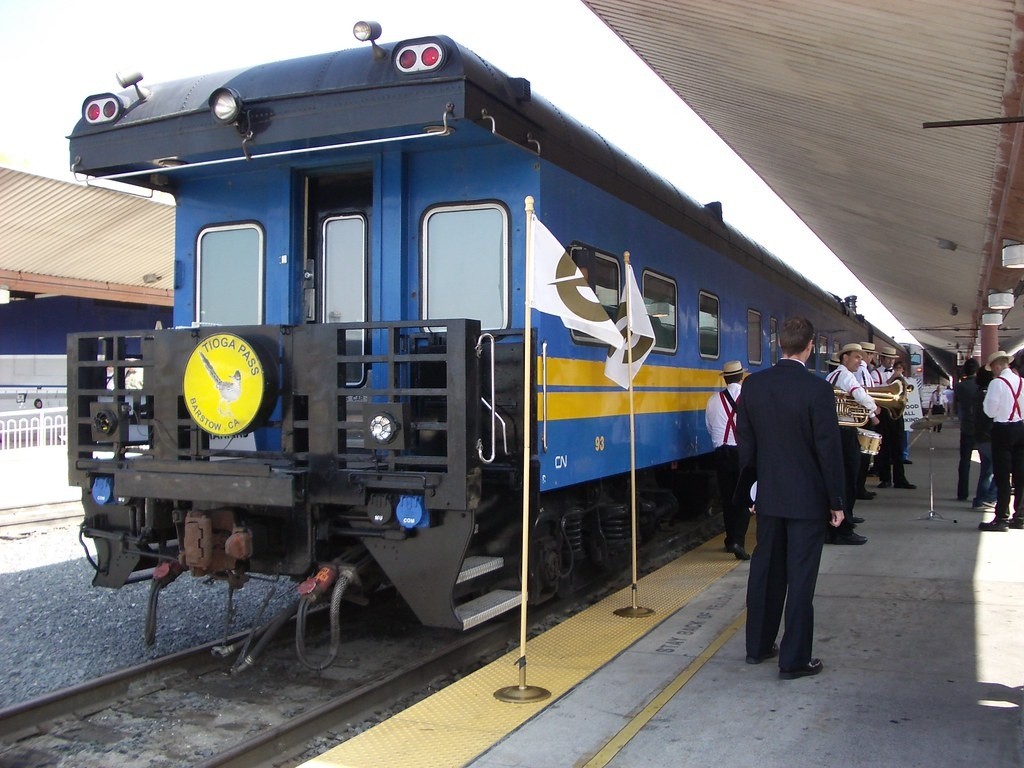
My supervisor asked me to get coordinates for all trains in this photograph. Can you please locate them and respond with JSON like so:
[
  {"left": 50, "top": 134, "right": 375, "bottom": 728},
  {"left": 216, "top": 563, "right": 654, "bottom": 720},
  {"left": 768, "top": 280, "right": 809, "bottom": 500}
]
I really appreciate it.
[{"left": 66, "top": 25, "right": 950, "bottom": 678}]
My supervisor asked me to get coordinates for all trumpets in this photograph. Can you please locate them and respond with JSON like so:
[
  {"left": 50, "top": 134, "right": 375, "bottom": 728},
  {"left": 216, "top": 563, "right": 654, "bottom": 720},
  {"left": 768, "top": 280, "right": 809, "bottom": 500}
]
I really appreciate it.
[{"left": 891, "top": 364, "right": 914, "bottom": 393}]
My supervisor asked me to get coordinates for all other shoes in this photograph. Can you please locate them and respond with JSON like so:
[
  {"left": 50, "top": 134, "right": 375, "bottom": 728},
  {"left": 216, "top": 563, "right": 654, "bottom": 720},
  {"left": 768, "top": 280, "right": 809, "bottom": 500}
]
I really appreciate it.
[
  {"left": 865, "top": 467, "right": 879, "bottom": 477},
  {"left": 972, "top": 500, "right": 997, "bottom": 511},
  {"left": 902, "top": 458, "right": 914, "bottom": 466}
]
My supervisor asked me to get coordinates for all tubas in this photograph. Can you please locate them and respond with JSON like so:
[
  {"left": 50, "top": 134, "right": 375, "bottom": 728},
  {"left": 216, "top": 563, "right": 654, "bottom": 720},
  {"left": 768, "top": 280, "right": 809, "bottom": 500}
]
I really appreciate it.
[{"left": 834, "top": 376, "right": 908, "bottom": 427}]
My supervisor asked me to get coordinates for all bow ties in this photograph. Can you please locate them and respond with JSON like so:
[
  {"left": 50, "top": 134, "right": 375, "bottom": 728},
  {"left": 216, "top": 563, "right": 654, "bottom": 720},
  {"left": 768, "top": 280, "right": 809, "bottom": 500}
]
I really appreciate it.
[{"left": 884, "top": 368, "right": 893, "bottom": 372}]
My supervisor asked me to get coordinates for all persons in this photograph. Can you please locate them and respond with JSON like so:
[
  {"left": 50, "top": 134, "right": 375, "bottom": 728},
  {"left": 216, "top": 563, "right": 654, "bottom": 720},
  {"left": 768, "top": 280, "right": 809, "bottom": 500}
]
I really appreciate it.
[
  {"left": 942, "top": 385, "right": 954, "bottom": 416},
  {"left": 823, "top": 340, "right": 918, "bottom": 544},
  {"left": 929, "top": 385, "right": 948, "bottom": 433},
  {"left": 106, "top": 358, "right": 144, "bottom": 389},
  {"left": 955, "top": 349, "right": 1024, "bottom": 532},
  {"left": 705, "top": 360, "right": 752, "bottom": 560},
  {"left": 736, "top": 316, "right": 848, "bottom": 680}
]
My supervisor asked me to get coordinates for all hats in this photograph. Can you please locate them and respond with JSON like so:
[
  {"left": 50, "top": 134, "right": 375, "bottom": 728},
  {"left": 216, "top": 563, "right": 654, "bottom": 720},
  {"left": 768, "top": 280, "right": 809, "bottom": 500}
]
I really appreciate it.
[
  {"left": 719, "top": 360, "right": 748, "bottom": 376},
  {"left": 876, "top": 346, "right": 900, "bottom": 358},
  {"left": 859, "top": 342, "right": 879, "bottom": 353},
  {"left": 985, "top": 351, "right": 1014, "bottom": 371},
  {"left": 825, "top": 352, "right": 841, "bottom": 366},
  {"left": 837, "top": 343, "right": 865, "bottom": 359}
]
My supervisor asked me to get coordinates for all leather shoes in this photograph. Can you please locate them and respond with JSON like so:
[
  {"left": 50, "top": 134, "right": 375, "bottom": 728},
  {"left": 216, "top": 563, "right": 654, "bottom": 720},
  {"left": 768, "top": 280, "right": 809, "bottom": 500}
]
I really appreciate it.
[
  {"left": 852, "top": 516, "right": 864, "bottom": 523},
  {"left": 723, "top": 536, "right": 731, "bottom": 552},
  {"left": 826, "top": 531, "right": 867, "bottom": 546},
  {"left": 876, "top": 481, "right": 892, "bottom": 488},
  {"left": 746, "top": 642, "right": 778, "bottom": 665},
  {"left": 778, "top": 657, "right": 822, "bottom": 681},
  {"left": 856, "top": 490, "right": 877, "bottom": 499},
  {"left": 893, "top": 480, "right": 918, "bottom": 489},
  {"left": 1008, "top": 518, "right": 1024, "bottom": 529},
  {"left": 978, "top": 518, "right": 1009, "bottom": 532},
  {"left": 732, "top": 543, "right": 750, "bottom": 560}
]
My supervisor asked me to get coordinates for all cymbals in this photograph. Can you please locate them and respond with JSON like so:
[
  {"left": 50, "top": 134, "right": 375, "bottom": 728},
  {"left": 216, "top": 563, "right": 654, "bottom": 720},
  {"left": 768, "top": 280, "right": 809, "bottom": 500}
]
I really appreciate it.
[{"left": 910, "top": 415, "right": 951, "bottom": 430}]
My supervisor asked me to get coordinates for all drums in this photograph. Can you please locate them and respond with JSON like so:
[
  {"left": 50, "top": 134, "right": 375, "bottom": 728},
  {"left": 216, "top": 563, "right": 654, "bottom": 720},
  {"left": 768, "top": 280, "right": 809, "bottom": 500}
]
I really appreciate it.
[{"left": 855, "top": 427, "right": 882, "bottom": 455}]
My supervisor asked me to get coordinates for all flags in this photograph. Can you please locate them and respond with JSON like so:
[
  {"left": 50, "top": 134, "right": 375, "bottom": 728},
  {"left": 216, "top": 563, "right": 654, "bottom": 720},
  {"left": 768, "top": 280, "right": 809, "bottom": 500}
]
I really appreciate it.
[
  {"left": 529, "top": 215, "right": 624, "bottom": 348},
  {"left": 605, "top": 266, "right": 655, "bottom": 391}
]
